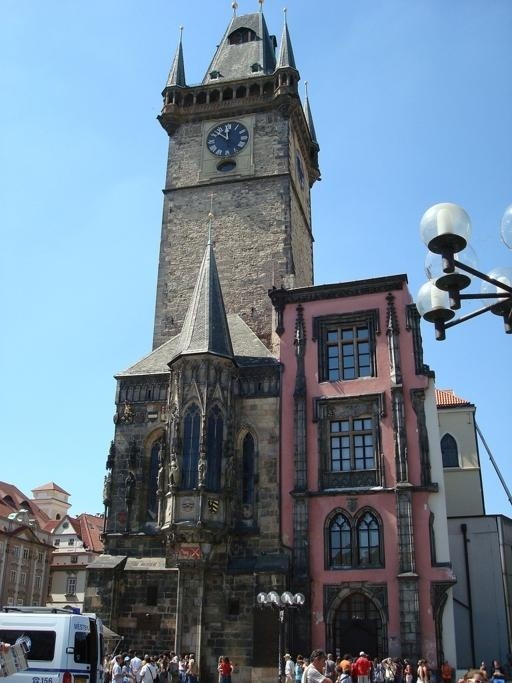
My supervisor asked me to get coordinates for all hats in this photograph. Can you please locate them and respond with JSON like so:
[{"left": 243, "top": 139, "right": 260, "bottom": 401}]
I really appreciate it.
[
  {"left": 360, "top": 651, "right": 365, "bottom": 655},
  {"left": 283, "top": 654, "right": 291, "bottom": 659},
  {"left": 124, "top": 656, "right": 131, "bottom": 661}
]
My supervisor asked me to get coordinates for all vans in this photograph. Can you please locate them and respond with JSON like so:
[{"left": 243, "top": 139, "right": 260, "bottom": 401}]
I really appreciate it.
[{"left": 0, "top": 605, "right": 105, "bottom": 683}]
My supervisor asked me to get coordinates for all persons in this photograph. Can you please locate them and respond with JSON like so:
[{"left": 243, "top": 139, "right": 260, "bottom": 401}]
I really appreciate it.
[
  {"left": 217, "top": 656, "right": 233, "bottom": 683},
  {"left": 284, "top": 650, "right": 430, "bottom": 683},
  {"left": 104, "top": 650, "right": 199, "bottom": 683},
  {"left": 102, "top": 439, "right": 234, "bottom": 504},
  {"left": 440, "top": 659, "right": 506, "bottom": 683}
]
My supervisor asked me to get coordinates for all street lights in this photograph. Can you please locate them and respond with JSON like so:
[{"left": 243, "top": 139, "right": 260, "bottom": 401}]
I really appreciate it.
[
  {"left": 418, "top": 201, "right": 511, "bottom": 340},
  {"left": 256, "top": 589, "right": 309, "bottom": 682}
]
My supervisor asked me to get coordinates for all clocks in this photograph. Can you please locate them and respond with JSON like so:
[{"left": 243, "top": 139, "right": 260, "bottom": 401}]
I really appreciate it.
[{"left": 204, "top": 120, "right": 251, "bottom": 159}]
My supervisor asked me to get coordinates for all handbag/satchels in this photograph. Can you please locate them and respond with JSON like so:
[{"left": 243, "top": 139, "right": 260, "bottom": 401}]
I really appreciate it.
[{"left": 153, "top": 674, "right": 160, "bottom": 683}]
